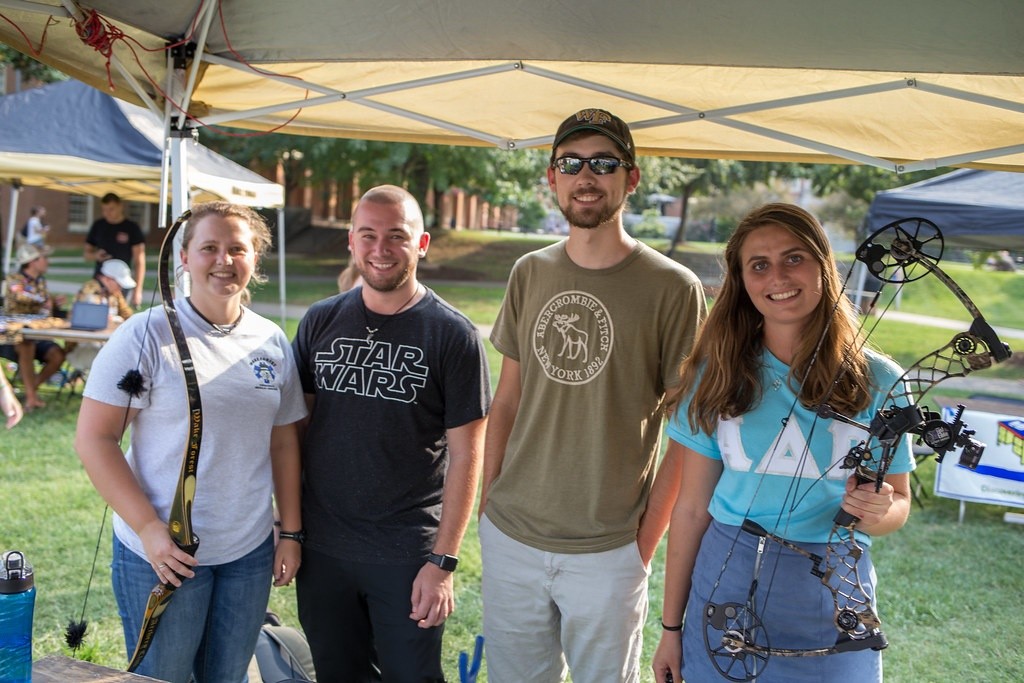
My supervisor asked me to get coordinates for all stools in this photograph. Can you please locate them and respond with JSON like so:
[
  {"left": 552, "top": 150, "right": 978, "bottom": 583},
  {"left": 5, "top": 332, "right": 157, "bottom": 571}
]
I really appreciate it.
[{"left": 910, "top": 441, "right": 935, "bottom": 508}]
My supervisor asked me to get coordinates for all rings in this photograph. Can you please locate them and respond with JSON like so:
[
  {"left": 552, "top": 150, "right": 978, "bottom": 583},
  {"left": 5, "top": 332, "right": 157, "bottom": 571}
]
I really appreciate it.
[{"left": 158, "top": 562, "right": 166, "bottom": 569}]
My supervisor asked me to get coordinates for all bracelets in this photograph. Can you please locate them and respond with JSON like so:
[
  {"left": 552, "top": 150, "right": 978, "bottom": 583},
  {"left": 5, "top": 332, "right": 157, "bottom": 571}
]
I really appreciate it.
[
  {"left": 0, "top": 383, "right": 6, "bottom": 389},
  {"left": 477, "top": 109, "right": 709, "bottom": 683},
  {"left": 662, "top": 622, "right": 683, "bottom": 631},
  {"left": 279, "top": 529, "right": 304, "bottom": 544}
]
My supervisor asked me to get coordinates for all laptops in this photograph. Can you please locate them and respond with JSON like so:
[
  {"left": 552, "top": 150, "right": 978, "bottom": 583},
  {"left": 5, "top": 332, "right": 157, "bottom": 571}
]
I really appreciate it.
[{"left": 62, "top": 301, "right": 108, "bottom": 331}]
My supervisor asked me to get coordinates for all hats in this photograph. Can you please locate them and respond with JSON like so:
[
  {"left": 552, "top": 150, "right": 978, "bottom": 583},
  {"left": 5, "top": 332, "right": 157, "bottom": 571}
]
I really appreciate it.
[
  {"left": 100, "top": 257, "right": 137, "bottom": 288},
  {"left": 551, "top": 107, "right": 637, "bottom": 162},
  {"left": 13, "top": 244, "right": 55, "bottom": 274}
]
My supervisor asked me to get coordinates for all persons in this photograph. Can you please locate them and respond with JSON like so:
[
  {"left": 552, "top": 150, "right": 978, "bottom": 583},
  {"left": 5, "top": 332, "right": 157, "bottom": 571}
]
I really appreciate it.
[
  {"left": 290, "top": 184, "right": 491, "bottom": 683},
  {"left": 652, "top": 202, "right": 917, "bottom": 683},
  {"left": 65, "top": 259, "right": 137, "bottom": 370},
  {"left": 0, "top": 204, "right": 66, "bottom": 430},
  {"left": 82, "top": 193, "right": 147, "bottom": 310},
  {"left": 75, "top": 200, "right": 310, "bottom": 683}
]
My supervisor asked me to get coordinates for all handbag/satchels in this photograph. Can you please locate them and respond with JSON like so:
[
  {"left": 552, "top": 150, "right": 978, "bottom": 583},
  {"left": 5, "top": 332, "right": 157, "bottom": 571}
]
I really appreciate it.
[{"left": 21, "top": 224, "right": 27, "bottom": 238}]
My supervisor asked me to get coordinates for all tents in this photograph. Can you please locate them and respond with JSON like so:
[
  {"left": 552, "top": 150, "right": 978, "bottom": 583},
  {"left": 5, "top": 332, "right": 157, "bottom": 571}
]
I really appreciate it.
[
  {"left": 853, "top": 167, "right": 1024, "bottom": 322},
  {"left": 0, "top": 76, "right": 287, "bottom": 337}
]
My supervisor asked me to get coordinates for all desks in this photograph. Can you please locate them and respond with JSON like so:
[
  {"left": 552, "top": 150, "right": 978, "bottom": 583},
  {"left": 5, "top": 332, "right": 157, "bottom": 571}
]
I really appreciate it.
[
  {"left": 31, "top": 652, "right": 173, "bottom": 683},
  {"left": 0, "top": 316, "right": 127, "bottom": 406},
  {"left": 930, "top": 397, "right": 1024, "bottom": 524}
]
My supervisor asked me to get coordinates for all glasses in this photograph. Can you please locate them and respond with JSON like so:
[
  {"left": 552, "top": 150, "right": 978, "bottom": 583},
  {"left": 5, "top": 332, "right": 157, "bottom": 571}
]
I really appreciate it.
[{"left": 550, "top": 156, "right": 634, "bottom": 175}]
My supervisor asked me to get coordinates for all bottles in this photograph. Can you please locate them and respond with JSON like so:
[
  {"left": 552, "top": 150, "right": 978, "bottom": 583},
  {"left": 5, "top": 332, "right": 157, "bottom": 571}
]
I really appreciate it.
[{"left": 0, "top": 551, "right": 37, "bottom": 683}]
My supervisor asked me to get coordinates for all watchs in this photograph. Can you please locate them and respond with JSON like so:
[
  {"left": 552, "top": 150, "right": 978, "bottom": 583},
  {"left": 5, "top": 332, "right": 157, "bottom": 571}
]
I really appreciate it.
[{"left": 427, "top": 553, "right": 459, "bottom": 572}]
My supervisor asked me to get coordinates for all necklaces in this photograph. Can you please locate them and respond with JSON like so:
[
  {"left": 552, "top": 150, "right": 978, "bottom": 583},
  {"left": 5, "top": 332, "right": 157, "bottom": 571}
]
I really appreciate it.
[
  {"left": 362, "top": 284, "right": 419, "bottom": 344},
  {"left": 768, "top": 354, "right": 789, "bottom": 391}
]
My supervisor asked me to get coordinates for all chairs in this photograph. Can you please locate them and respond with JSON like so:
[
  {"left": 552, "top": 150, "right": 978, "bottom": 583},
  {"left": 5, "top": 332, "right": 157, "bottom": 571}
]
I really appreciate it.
[
  {"left": 53, "top": 311, "right": 87, "bottom": 405},
  {"left": 0, "top": 296, "right": 40, "bottom": 391}
]
